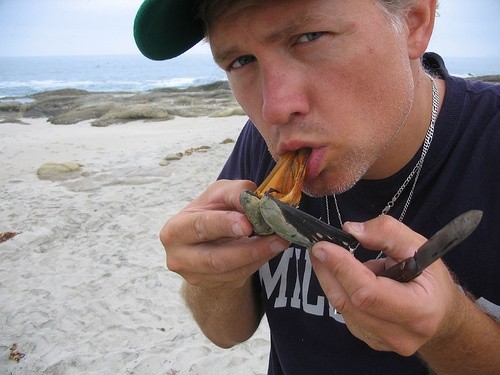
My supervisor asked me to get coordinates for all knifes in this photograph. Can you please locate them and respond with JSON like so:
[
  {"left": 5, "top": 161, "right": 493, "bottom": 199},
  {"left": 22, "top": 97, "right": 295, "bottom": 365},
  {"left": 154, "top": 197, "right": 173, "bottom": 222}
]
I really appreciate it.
[{"left": 376, "top": 208, "right": 483, "bottom": 283}]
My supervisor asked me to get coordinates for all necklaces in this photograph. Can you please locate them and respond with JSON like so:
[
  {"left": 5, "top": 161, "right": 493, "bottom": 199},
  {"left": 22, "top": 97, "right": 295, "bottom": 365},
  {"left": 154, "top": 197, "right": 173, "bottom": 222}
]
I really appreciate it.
[{"left": 325, "top": 73, "right": 440, "bottom": 317}]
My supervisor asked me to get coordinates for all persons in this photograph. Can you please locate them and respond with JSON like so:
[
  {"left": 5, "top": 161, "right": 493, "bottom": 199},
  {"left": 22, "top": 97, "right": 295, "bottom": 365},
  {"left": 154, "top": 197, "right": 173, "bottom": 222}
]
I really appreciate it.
[{"left": 133, "top": 0, "right": 500, "bottom": 375}]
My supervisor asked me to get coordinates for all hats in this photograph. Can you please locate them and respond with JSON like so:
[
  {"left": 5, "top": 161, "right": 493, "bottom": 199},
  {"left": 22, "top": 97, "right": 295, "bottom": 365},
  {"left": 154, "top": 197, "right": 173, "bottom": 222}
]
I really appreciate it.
[{"left": 133, "top": 1, "right": 207, "bottom": 61}]
